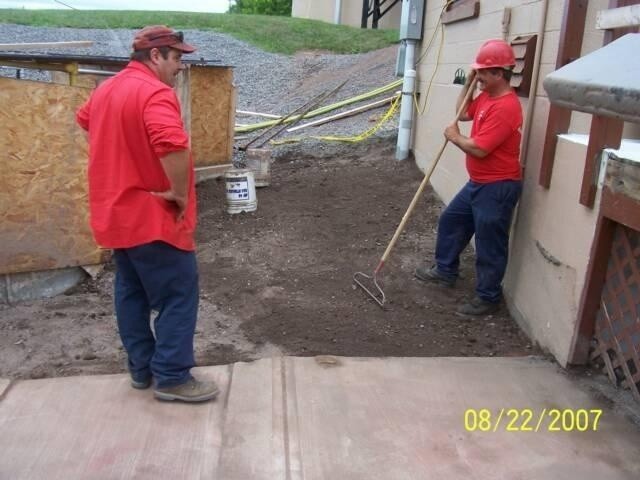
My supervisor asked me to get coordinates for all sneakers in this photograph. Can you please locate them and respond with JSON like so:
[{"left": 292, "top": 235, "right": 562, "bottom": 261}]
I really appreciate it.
[
  {"left": 131, "top": 367, "right": 151, "bottom": 388},
  {"left": 456, "top": 300, "right": 495, "bottom": 317},
  {"left": 154, "top": 376, "right": 219, "bottom": 402},
  {"left": 415, "top": 264, "right": 456, "bottom": 285}
]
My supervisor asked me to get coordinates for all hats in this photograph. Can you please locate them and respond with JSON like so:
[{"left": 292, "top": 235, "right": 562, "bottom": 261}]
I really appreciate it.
[{"left": 132, "top": 25, "right": 195, "bottom": 53}]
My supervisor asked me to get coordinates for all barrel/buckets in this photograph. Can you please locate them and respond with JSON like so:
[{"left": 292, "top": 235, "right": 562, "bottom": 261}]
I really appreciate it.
[
  {"left": 224, "top": 168, "right": 258, "bottom": 215},
  {"left": 247, "top": 148, "right": 272, "bottom": 187}
]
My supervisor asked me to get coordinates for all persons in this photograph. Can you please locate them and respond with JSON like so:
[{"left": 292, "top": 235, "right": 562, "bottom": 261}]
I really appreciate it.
[
  {"left": 76, "top": 22, "right": 221, "bottom": 402},
  {"left": 413, "top": 38, "right": 525, "bottom": 316}
]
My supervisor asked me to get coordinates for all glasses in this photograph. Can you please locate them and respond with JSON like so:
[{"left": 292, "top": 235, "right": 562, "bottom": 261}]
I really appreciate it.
[{"left": 149, "top": 31, "right": 183, "bottom": 41}]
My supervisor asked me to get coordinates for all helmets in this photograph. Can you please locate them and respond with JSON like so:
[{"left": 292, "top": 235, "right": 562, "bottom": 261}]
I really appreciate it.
[{"left": 470, "top": 40, "right": 515, "bottom": 69}]
